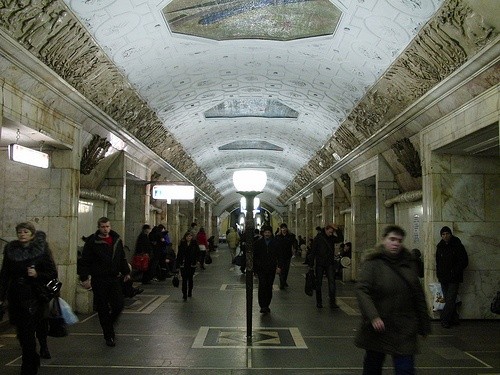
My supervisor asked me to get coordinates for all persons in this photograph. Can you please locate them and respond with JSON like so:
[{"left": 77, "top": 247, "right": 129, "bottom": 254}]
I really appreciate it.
[
  {"left": 175, "top": 230, "right": 200, "bottom": 300},
  {"left": 251, "top": 224, "right": 281, "bottom": 316},
  {"left": 124, "top": 222, "right": 216, "bottom": 297},
  {"left": 353, "top": 226, "right": 430, "bottom": 375},
  {"left": 226, "top": 222, "right": 351, "bottom": 290},
  {"left": 436, "top": 226, "right": 468, "bottom": 329},
  {"left": 79, "top": 217, "right": 130, "bottom": 346},
  {"left": 0, "top": 222, "right": 57, "bottom": 375},
  {"left": 411, "top": 248, "right": 424, "bottom": 278},
  {"left": 308, "top": 224, "right": 344, "bottom": 310}
]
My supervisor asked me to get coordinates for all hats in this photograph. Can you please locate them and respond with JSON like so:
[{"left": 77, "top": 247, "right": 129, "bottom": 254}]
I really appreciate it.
[{"left": 440, "top": 226, "right": 452, "bottom": 237}]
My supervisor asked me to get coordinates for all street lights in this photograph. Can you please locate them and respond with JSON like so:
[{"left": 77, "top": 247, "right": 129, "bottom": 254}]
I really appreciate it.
[{"left": 232, "top": 169, "right": 268, "bottom": 345}]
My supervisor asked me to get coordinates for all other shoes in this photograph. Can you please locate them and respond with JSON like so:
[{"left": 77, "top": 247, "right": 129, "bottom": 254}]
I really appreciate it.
[
  {"left": 188, "top": 291, "right": 192, "bottom": 297},
  {"left": 38, "top": 345, "right": 51, "bottom": 359},
  {"left": 240, "top": 268, "right": 245, "bottom": 274},
  {"left": 441, "top": 320, "right": 457, "bottom": 328},
  {"left": 128, "top": 287, "right": 144, "bottom": 299},
  {"left": 183, "top": 295, "right": 187, "bottom": 300},
  {"left": 316, "top": 303, "right": 323, "bottom": 308},
  {"left": 260, "top": 305, "right": 270, "bottom": 313},
  {"left": 105, "top": 333, "right": 117, "bottom": 346},
  {"left": 279, "top": 282, "right": 288, "bottom": 290},
  {"left": 330, "top": 303, "right": 339, "bottom": 309}
]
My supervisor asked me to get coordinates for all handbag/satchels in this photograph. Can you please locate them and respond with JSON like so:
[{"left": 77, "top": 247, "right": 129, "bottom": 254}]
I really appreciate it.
[
  {"left": 36, "top": 279, "right": 62, "bottom": 301},
  {"left": 48, "top": 296, "right": 80, "bottom": 338},
  {"left": 172, "top": 277, "right": 180, "bottom": 287},
  {"left": 132, "top": 255, "right": 150, "bottom": 268},
  {"left": 305, "top": 269, "right": 318, "bottom": 296},
  {"left": 231, "top": 255, "right": 245, "bottom": 266},
  {"left": 205, "top": 255, "right": 212, "bottom": 264}
]
